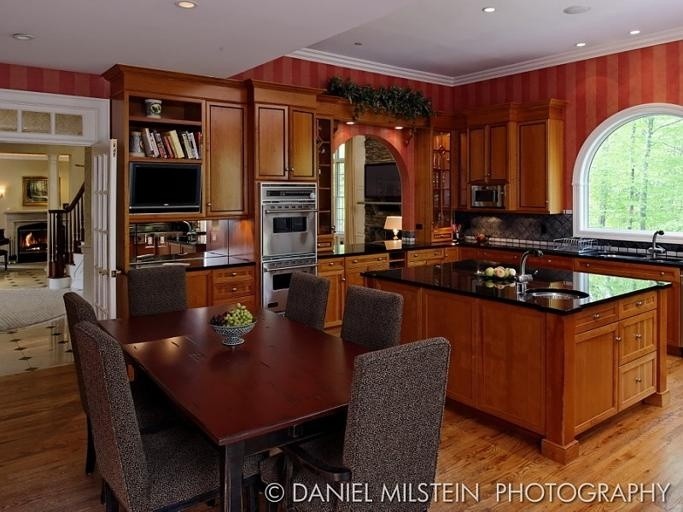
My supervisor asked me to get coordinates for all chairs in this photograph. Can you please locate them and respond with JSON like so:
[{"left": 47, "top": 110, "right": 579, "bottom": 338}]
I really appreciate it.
[
  {"left": 259, "top": 337, "right": 451, "bottom": 511},
  {"left": 63, "top": 292, "right": 180, "bottom": 504},
  {"left": 285, "top": 271, "right": 331, "bottom": 330},
  {"left": 72, "top": 320, "right": 259, "bottom": 511},
  {"left": 340, "top": 285, "right": 404, "bottom": 348},
  {"left": 127, "top": 265, "right": 188, "bottom": 317}
]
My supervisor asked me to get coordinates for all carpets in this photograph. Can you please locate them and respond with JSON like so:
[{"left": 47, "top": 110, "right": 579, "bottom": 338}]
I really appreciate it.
[{"left": 0, "top": 289, "right": 83, "bottom": 331}]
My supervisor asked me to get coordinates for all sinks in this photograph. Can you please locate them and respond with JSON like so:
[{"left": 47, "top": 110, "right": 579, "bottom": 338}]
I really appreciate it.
[
  {"left": 649, "top": 257, "right": 682, "bottom": 262},
  {"left": 597, "top": 251, "right": 647, "bottom": 258},
  {"left": 525, "top": 288, "right": 588, "bottom": 300}
]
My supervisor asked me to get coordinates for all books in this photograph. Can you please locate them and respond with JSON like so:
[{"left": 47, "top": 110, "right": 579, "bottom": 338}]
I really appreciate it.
[{"left": 141, "top": 128, "right": 202, "bottom": 160}]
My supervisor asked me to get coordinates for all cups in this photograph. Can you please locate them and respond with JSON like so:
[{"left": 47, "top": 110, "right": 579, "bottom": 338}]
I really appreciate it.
[{"left": 144, "top": 99, "right": 162, "bottom": 119}]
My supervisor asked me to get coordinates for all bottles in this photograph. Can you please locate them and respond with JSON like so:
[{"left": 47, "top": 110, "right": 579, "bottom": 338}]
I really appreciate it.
[{"left": 400, "top": 230, "right": 415, "bottom": 246}]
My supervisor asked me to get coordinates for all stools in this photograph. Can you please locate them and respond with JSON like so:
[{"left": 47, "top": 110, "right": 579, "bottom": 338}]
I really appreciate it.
[{"left": 0, "top": 250, "right": 8, "bottom": 271}]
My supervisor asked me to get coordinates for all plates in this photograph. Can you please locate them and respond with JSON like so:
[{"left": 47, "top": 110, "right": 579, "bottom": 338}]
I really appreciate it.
[{"left": 474, "top": 235, "right": 490, "bottom": 242}]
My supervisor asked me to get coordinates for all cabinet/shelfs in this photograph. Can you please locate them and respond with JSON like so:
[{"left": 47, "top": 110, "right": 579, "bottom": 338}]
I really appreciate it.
[
  {"left": 445, "top": 247, "right": 459, "bottom": 263},
  {"left": 574, "top": 301, "right": 618, "bottom": 437},
  {"left": 317, "top": 257, "right": 345, "bottom": 330},
  {"left": 117, "top": 271, "right": 213, "bottom": 319},
  {"left": 433, "top": 129, "right": 459, "bottom": 227},
  {"left": 345, "top": 253, "right": 389, "bottom": 295},
  {"left": 407, "top": 248, "right": 445, "bottom": 268},
  {"left": 316, "top": 114, "right": 335, "bottom": 255},
  {"left": 213, "top": 266, "right": 261, "bottom": 308},
  {"left": 460, "top": 248, "right": 476, "bottom": 262},
  {"left": 467, "top": 121, "right": 519, "bottom": 185},
  {"left": 619, "top": 291, "right": 658, "bottom": 412},
  {"left": 207, "top": 98, "right": 253, "bottom": 220},
  {"left": 476, "top": 248, "right": 683, "bottom": 347},
  {"left": 111, "top": 90, "right": 207, "bottom": 222},
  {"left": 519, "top": 119, "right": 565, "bottom": 211},
  {"left": 253, "top": 102, "right": 316, "bottom": 180}
]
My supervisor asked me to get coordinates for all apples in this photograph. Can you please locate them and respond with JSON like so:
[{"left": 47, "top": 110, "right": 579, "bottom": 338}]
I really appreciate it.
[{"left": 485, "top": 267, "right": 516, "bottom": 278}]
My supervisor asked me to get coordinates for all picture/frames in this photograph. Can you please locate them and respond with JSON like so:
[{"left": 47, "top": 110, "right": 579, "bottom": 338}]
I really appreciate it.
[{"left": 22, "top": 176, "right": 61, "bottom": 206}]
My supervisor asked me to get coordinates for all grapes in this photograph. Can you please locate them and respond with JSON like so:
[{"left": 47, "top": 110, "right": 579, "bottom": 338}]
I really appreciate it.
[
  {"left": 210, "top": 311, "right": 228, "bottom": 325},
  {"left": 224, "top": 302, "right": 253, "bottom": 326}
]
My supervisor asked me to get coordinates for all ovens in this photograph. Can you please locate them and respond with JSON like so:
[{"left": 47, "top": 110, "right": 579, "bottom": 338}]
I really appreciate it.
[
  {"left": 471, "top": 185, "right": 501, "bottom": 208},
  {"left": 259, "top": 182, "right": 317, "bottom": 318}
]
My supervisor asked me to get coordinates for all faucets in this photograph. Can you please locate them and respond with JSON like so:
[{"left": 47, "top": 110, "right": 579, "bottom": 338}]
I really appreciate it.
[
  {"left": 516, "top": 249, "right": 544, "bottom": 295},
  {"left": 648, "top": 230, "right": 666, "bottom": 259}
]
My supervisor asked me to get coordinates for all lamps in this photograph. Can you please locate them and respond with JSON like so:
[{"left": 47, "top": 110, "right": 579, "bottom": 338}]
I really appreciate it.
[
  {"left": 383, "top": 216, "right": 402, "bottom": 236},
  {"left": 383, "top": 236, "right": 402, "bottom": 250}
]
